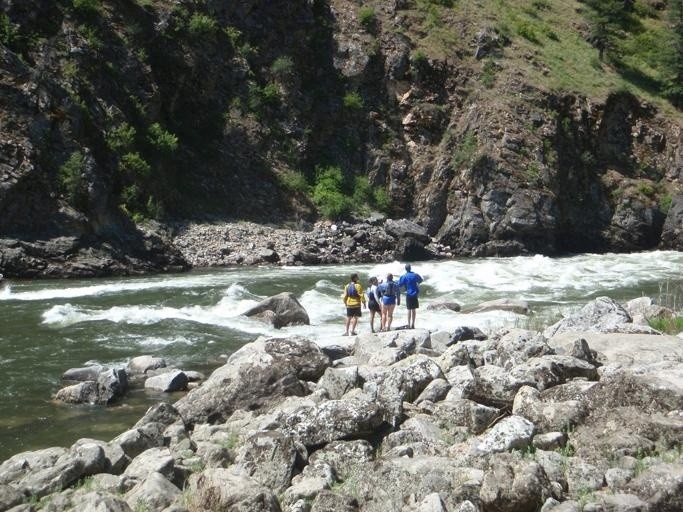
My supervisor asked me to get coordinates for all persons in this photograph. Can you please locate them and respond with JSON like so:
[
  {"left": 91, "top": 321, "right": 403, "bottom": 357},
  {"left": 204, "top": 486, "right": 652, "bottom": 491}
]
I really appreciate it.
[{"left": 342, "top": 264, "right": 423, "bottom": 336}]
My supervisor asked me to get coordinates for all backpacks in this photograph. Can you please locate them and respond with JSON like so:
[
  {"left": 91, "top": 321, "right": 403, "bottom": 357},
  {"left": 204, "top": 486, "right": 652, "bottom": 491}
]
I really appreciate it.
[
  {"left": 384, "top": 281, "right": 395, "bottom": 296},
  {"left": 347, "top": 282, "right": 359, "bottom": 297}
]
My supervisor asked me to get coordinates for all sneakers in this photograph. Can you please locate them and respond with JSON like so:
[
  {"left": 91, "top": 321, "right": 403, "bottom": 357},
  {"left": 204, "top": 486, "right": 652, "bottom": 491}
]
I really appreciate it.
[
  {"left": 372, "top": 328, "right": 392, "bottom": 333},
  {"left": 407, "top": 326, "right": 415, "bottom": 329},
  {"left": 342, "top": 332, "right": 357, "bottom": 336}
]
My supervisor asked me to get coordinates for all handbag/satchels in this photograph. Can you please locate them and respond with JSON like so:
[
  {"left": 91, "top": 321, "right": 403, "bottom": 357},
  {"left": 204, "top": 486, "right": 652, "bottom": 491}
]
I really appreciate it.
[{"left": 368, "top": 292, "right": 375, "bottom": 301}]
